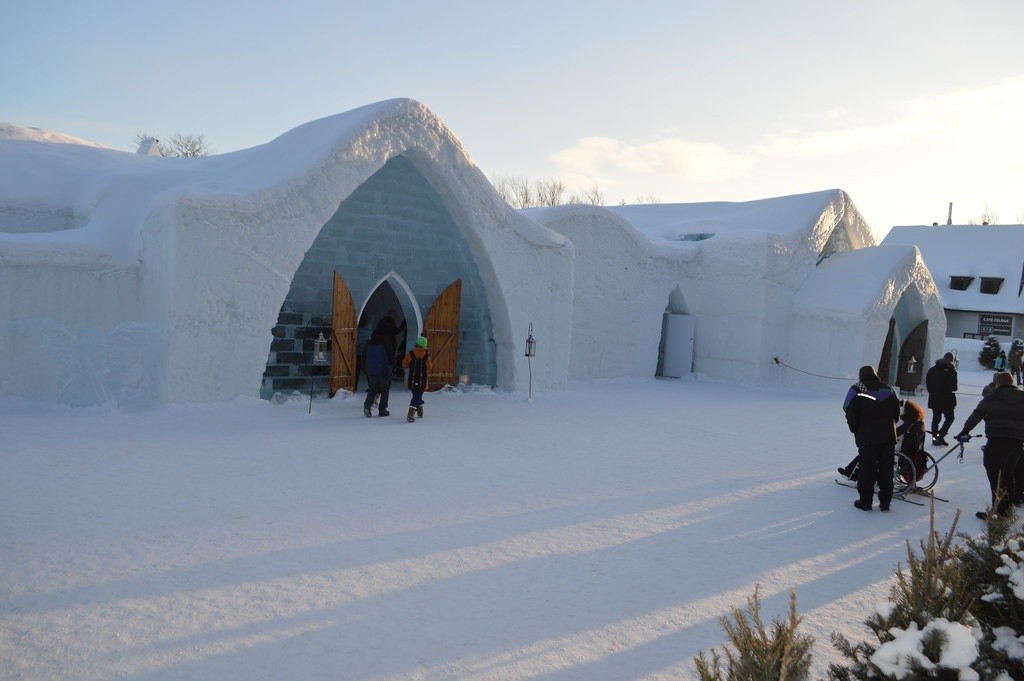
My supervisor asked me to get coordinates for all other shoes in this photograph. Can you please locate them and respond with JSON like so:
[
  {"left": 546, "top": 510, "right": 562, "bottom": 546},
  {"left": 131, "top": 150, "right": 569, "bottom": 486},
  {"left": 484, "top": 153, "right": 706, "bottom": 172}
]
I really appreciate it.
[
  {"left": 854, "top": 501, "right": 872, "bottom": 510},
  {"left": 363, "top": 409, "right": 371, "bottom": 417},
  {"left": 932, "top": 436, "right": 948, "bottom": 446},
  {"left": 378, "top": 410, "right": 389, "bottom": 416},
  {"left": 879, "top": 506, "right": 889, "bottom": 512},
  {"left": 838, "top": 467, "right": 850, "bottom": 477},
  {"left": 1017, "top": 381, "right": 1023, "bottom": 385},
  {"left": 975, "top": 512, "right": 990, "bottom": 520}
]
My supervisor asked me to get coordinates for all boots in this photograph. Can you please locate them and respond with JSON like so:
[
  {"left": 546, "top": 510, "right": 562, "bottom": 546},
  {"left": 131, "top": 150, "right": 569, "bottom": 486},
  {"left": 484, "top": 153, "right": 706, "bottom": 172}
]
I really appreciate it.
[{"left": 407, "top": 405, "right": 423, "bottom": 422}]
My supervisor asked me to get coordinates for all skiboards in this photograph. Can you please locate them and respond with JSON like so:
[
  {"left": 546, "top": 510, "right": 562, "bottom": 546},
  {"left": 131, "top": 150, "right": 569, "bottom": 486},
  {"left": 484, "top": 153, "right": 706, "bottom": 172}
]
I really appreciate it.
[{"left": 835, "top": 477, "right": 950, "bottom": 506}]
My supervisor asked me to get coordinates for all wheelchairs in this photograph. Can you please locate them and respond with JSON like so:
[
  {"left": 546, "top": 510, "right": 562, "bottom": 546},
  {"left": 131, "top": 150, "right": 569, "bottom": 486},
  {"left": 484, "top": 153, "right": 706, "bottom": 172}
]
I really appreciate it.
[{"left": 841, "top": 429, "right": 939, "bottom": 497}]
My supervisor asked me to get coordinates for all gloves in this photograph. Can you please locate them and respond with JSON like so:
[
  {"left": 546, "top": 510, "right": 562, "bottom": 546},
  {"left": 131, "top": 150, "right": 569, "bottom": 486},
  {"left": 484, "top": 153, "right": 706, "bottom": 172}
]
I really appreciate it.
[{"left": 954, "top": 430, "right": 968, "bottom": 442}]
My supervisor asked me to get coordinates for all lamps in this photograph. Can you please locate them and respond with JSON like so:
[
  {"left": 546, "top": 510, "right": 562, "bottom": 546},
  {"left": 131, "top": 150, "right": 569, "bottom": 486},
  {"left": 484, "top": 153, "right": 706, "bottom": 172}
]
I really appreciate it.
[
  {"left": 950, "top": 347, "right": 960, "bottom": 371},
  {"left": 304, "top": 317, "right": 328, "bottom": 417},
  {"left": 906, "top": 356, "right": 917, "bottom": 397},
  {"left": 525, "top": 322, "right": 537, "bottom": 400}
]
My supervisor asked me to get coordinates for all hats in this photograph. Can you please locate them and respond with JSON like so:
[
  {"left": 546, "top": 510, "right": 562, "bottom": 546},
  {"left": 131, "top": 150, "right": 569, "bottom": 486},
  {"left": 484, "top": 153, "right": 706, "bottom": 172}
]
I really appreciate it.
[
  {"left": 1002, "top": 350, "right": 1005, "bottom": 354},
  {"left": 859, "top": 365, "right": 875, "bottom": 376},
  {"left": 416, "top": 337, "right": 427, "bottom": 348},
  {"left": 943, "top": 352, "right": 953, "bottom": 363},
  {"left": 993, "top": 372, "right": 1013, "bottom": 384}
]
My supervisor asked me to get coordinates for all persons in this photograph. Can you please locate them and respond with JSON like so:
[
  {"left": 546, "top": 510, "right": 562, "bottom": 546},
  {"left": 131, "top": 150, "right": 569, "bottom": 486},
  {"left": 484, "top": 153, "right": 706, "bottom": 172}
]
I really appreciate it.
[
  {"left": 925, "top": 352, "right": 958, "bottom": 446},
  {"left": 957, "top": 338, "right": 1024, "bottom": 520},
  {"left": 843, "top": 366, "right": 926, "bottom": 512},
  {"left": 402, "top": 337, "right": 432, "bottom": 423},
  {"left": 355, "top": 310, "right": 407, "bottom": 416}
]
